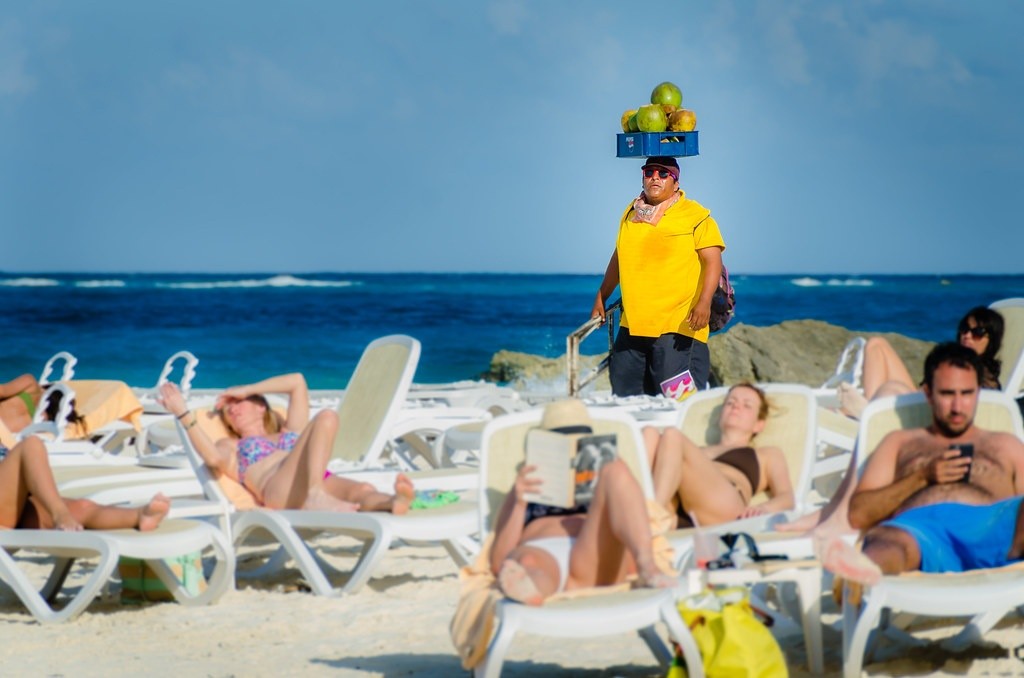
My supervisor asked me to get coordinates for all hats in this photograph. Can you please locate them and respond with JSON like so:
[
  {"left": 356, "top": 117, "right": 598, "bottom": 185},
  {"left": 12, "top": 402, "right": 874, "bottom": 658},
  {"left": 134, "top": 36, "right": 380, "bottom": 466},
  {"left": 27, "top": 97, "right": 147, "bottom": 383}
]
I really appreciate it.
[
  {"left": 642, "top": 155, "right": 680, "bottom": 176},
  {"left": 532, "top": 399, "right": 596, "bottom": 458}
]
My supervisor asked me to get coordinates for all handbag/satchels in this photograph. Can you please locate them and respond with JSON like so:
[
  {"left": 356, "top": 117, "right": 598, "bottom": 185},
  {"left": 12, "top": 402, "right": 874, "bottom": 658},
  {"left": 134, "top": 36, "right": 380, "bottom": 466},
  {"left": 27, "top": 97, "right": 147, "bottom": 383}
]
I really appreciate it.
[
  {"left": 664, "top": 588, "right": 789, "bottom": 678},
  {"left": 120, "top": 553, "right": 206, "bottom": 604}
]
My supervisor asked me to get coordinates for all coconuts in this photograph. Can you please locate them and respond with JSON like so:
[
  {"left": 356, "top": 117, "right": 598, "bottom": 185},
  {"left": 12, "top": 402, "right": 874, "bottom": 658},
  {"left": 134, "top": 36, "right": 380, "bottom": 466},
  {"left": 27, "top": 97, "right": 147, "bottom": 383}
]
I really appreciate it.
[{"left": 620, "top": 82, "right": 697, "bottom": 142}]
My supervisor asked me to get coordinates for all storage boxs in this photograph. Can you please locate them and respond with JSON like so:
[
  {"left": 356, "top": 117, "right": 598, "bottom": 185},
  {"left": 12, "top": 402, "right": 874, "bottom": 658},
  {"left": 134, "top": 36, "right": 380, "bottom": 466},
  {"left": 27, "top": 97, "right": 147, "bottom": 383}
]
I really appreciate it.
[{"left": 617, "top": 130, "right": 700, "bottom": 158}]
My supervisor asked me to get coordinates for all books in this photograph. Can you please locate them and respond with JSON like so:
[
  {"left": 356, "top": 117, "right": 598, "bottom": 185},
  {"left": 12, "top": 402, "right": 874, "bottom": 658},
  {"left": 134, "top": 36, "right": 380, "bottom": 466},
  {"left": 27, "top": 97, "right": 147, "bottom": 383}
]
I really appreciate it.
[{"left": 522, "top": 425, "right": 616, "bottom": 510}]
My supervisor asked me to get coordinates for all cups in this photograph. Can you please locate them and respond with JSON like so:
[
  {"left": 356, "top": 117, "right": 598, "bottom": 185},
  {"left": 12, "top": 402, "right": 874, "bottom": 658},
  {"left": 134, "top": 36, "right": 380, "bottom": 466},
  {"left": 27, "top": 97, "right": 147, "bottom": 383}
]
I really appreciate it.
[{"left": 693, "top": 533, "right": 718, "bottom": 568}]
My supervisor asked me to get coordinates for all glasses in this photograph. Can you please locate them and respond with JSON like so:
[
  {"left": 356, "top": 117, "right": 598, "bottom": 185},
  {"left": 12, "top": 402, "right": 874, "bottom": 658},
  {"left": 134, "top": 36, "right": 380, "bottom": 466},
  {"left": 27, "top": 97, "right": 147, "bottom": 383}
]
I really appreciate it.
[
  {"left": 642, "top": 167, "right": 675, "bottom": 179},
  {"left": 961, "top": 321, "right": 988, "bottom": 340}
]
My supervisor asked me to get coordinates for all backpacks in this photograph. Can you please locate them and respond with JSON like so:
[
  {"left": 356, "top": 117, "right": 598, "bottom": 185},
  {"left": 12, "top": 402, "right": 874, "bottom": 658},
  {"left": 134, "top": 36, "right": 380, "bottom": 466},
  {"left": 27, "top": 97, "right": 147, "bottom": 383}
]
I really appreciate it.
[{"left": 625, "top": 205, "right": 735, "bottom": 332}]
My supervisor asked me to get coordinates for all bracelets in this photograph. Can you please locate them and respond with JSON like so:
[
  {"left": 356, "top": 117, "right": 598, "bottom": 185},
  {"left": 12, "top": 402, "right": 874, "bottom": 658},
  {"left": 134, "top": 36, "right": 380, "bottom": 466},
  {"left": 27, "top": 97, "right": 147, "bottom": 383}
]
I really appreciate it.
[
  {"left": 185, "top": 419, "right": 198, "bottom": 429},
  {"left": 178, "top": 410, "right": 189, "bottom": 419}
]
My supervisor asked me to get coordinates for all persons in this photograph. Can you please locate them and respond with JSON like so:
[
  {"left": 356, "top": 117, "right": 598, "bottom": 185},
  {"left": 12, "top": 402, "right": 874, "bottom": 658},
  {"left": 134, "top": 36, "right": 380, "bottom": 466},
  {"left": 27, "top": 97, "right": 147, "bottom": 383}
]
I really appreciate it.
[
  {"left": 0, "top": 436, "right": 168, "bottom": 531},
  {"left": 0, "top": 373, "right": 89, "bottom": 452},
  {"left": 151, "top": 371, "right": 417, "bottom": 514},
  {"left": 811, "top": 336, "right": 1024, "bottom": 585},
  {"left": 837, "top": 303, "right": 1004, "bottom": 424},
  {"left": 486, "top": 394, "right": 681, "bottom": 605},
  {"left": 588, "top": 157, "right": 725, "bottom": 400},
  {"left": 640, "top": 380, "right": 796, "bottom": 528}
]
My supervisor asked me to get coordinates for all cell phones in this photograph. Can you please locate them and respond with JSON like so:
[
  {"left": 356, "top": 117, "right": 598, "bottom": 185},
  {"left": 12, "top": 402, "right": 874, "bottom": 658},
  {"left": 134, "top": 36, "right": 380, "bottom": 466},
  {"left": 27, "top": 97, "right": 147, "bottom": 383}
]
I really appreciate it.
[{"left": 948, "top": 442, "right": 974, "bottom": 482}]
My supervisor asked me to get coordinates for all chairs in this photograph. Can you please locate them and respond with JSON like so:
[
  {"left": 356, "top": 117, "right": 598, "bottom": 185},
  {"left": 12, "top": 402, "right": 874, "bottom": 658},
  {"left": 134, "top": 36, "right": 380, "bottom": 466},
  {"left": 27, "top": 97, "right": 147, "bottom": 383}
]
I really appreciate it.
[{"left": 2, "top": 299, "right": 1024, "bottom": 678}]
